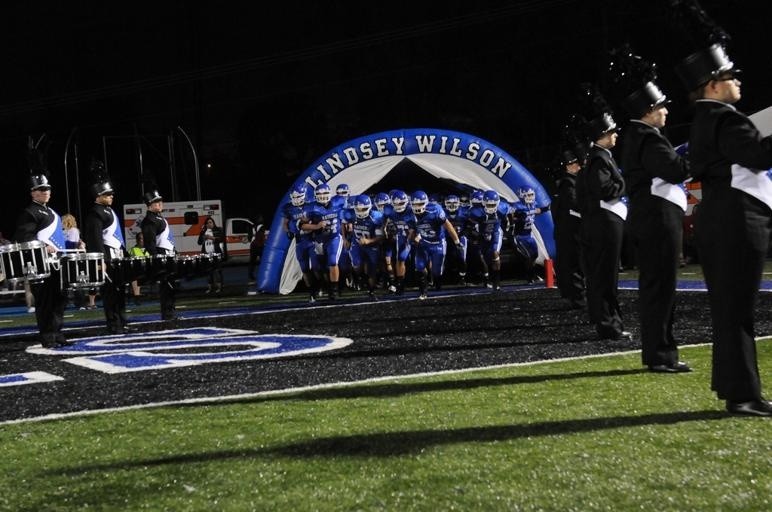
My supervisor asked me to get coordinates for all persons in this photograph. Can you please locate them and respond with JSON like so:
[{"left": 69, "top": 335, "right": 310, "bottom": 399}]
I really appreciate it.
[
  {"left": 282, "top": 184, "right": 555, "bottom": 302},
  {"left": 197, "top": 217, "right": 227, "bottom": 297},
  {"left": 247, "top": 215, "right": 266, "bottom": 296},
  {"left": 16, "top": 175, "right": 177, "bottom": 347},
  {"left": 556, "top": 44, "right": 771, "bottom": 416}
]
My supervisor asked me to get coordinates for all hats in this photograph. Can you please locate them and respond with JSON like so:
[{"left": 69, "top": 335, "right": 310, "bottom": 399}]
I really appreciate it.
[
  {"left": 560, "top": 43, "right": 742, "bottom": 166},
  {"left": 31, "top": 175, "right": 52, "bottom": 190},
  {"left": 144, "top": 190, "right": 162, "bottom": 207},
  {"left": 94, "top": 182, "right": 113, "bottom": 196}
]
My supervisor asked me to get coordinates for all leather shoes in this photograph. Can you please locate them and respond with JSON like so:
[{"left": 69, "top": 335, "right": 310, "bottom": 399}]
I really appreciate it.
[
  {"left": 648, "top": 361, "right": 687, "bottom": 372},
  {"left": 726, "top": 396, "right": 772, "bottom": 416}
]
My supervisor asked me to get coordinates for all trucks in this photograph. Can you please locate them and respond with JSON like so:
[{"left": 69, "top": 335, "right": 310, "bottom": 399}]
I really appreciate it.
[
  {"left": 123, "top": 199, "right": 270, "bottom": 266},
  {"left": 673, "top": 142, "right": 703, "bottom": 238}
]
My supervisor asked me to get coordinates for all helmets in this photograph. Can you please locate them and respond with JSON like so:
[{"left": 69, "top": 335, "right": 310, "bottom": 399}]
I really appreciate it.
[{"left": 289, "top": 184, "right": 536, "bottom": 219}]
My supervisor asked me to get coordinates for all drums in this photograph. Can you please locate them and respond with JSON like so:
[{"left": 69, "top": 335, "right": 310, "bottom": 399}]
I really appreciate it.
[
  {"left": 113, "top": 252, "right": 222, "bottom": 285},
  {"left": 60, "top": 252, "right": 105, "bottom": 292},
  {"left": 0, "top": 238, "right": 51, "bottom": 288}
]
